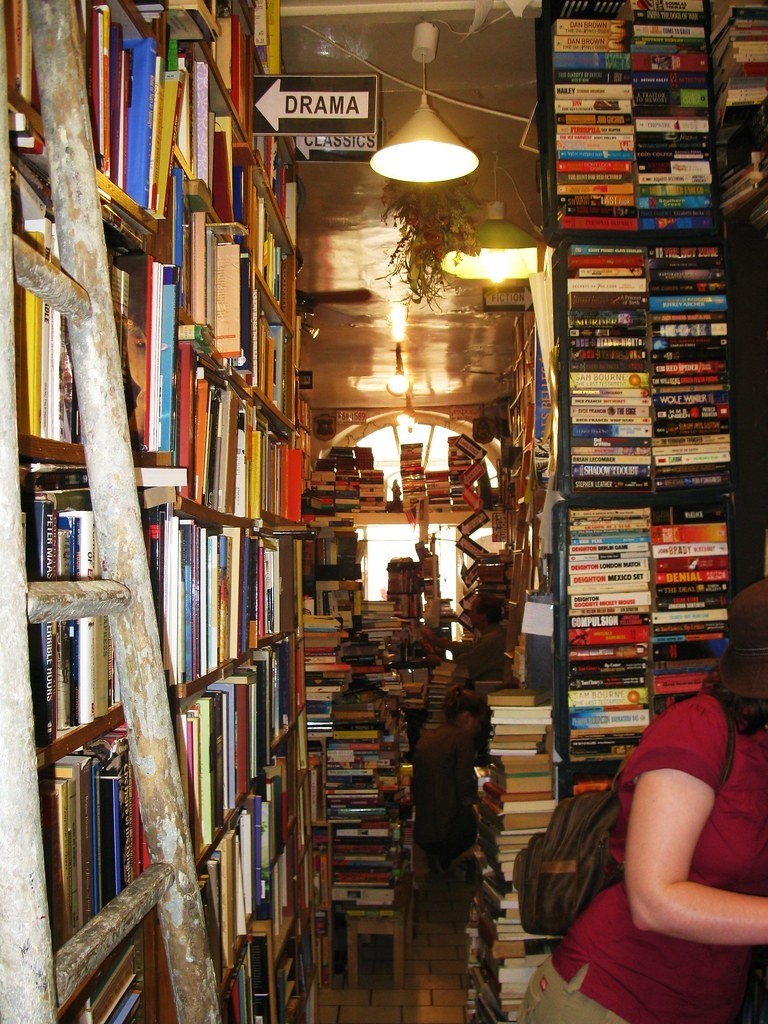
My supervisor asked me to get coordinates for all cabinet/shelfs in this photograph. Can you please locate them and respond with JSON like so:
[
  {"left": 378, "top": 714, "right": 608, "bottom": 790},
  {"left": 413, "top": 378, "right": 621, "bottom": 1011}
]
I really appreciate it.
[{"left": 4, "top": 0, "right": 318, "bottom": 1023}]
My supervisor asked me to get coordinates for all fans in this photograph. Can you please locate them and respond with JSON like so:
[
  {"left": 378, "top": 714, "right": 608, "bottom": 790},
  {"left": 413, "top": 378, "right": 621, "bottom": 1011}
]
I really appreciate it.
[{"left": 296, "top": 250, "right": 373, "bottom": 317}]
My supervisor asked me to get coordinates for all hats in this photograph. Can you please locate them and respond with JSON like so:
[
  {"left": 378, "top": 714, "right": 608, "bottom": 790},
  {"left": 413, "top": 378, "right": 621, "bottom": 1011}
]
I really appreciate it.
[{"left": 720, "top": 579, "right": 768, "bottom": 699}]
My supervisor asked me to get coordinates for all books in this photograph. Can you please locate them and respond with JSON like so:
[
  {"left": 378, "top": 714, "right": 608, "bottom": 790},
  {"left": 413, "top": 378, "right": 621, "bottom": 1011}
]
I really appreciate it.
[
  {"left": 468, "top": 1, "right": 768, "bottom": 1024},
  {"left": 3, "top": 0, "right": 470, "bottom": 1024}
]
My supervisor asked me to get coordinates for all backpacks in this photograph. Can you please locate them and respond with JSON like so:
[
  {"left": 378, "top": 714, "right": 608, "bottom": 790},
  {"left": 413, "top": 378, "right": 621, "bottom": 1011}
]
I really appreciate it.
[{"left": 513, "top": 747, "right": 634, "bottom": 935}]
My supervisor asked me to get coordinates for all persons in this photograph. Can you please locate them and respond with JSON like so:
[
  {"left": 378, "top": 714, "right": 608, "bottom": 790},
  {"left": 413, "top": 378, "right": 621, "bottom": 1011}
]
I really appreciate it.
[
  {"left": 415, "top": 591, "right": 507, "bottom": 693},
  {"left": 405, "top": 683, "right": 492, "bottom": 888},
  {"left": 519, "top": 576, "right": 768, "bottom": 1024}
]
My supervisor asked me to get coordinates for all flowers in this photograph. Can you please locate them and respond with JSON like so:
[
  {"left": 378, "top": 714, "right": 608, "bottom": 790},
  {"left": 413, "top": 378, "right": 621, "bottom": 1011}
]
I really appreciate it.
[{"left": 379, "top": 173, "right": 482, "bottom": 315}]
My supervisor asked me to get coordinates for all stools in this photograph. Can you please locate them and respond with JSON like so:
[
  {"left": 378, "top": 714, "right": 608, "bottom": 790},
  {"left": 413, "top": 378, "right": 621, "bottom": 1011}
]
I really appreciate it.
[{"left": 344, "top": 873, "right": 415, "bottom": 990}]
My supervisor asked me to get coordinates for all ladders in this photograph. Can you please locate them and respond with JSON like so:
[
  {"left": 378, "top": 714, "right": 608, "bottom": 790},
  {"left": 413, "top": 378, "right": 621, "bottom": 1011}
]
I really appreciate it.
[{"left": 0, "top": 12, "right": 225, "bottom": 1024}]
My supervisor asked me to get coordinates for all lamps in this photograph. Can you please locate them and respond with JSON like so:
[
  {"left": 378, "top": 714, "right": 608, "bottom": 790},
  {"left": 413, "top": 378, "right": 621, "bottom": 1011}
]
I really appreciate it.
[
  {"left": 369, "top": 17, "right": 481, "bottom": 182},
  {"left": 443, "top": 153, "right": 546, "bottom": 281},
  {"left": 302, "top": 323, "right": 321, "bottom": 340},
  {"left": 387, "top": 343, "right": 418, "bottom": 435}
]
